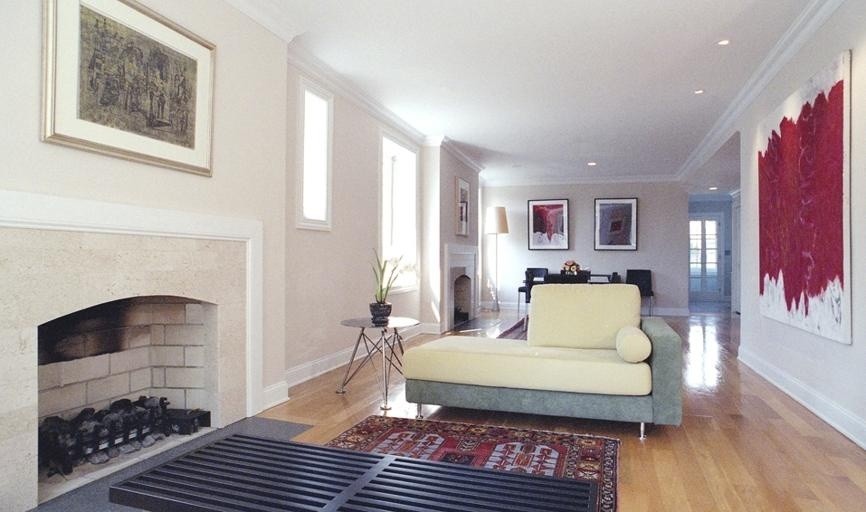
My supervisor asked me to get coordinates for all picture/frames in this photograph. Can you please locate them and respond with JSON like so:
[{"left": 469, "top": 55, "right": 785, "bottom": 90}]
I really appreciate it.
[
  {"left": 39, "top": 0, "right": 221, "bottom": 181},
  {"left": 525, "top": 197, "right": 571, "bottom": 252},
  {"left": 593, "top": 196, "right": 640, "bottom": 251},
  {"left": 454, "top": 173, "right": 472, "bottom": 239}
]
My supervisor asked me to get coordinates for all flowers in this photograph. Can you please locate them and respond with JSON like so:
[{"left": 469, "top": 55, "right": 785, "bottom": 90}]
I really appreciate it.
[{"left": 560, "top": 259, "right": 581, "bottom": 274}]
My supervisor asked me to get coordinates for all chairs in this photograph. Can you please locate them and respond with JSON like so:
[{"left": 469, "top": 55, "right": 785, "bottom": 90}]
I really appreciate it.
[{"left": 515, "top": 266, "right": 656, "bottom": 333}]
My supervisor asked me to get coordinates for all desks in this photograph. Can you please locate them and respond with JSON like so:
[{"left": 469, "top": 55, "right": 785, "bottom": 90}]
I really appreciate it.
[
  {"left": 334, "top": 315, "right": 421, "bottom": 412},
  {"left": 110, "top": 430, "right": 601, "bottom": 512}
]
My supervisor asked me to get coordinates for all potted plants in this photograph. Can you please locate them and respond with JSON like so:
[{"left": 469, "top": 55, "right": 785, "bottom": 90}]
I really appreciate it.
[{"left": 366, "top": 240, "right": 413, "bottom": 328}]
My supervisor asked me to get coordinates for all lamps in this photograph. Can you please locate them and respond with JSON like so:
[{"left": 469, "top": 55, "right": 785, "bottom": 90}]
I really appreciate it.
[{"left": 483, "top": 203, "right": 511, "bottom": 314}]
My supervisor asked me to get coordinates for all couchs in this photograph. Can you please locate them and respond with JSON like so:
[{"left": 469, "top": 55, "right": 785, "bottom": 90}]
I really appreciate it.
[{"left": 400, "top": 275, "right": 687, "bottom": 441}]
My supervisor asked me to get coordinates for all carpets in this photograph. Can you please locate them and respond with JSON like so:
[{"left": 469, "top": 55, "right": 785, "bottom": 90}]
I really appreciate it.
[
  {"left": 22, "top": 415, "right": 313, "bottom": 510},
  {"left": 323, "top": 413, "right": 621, "bottom": 512},
  {"left": 496, "top": 311, "right": 530, "bottom": 340}
]
